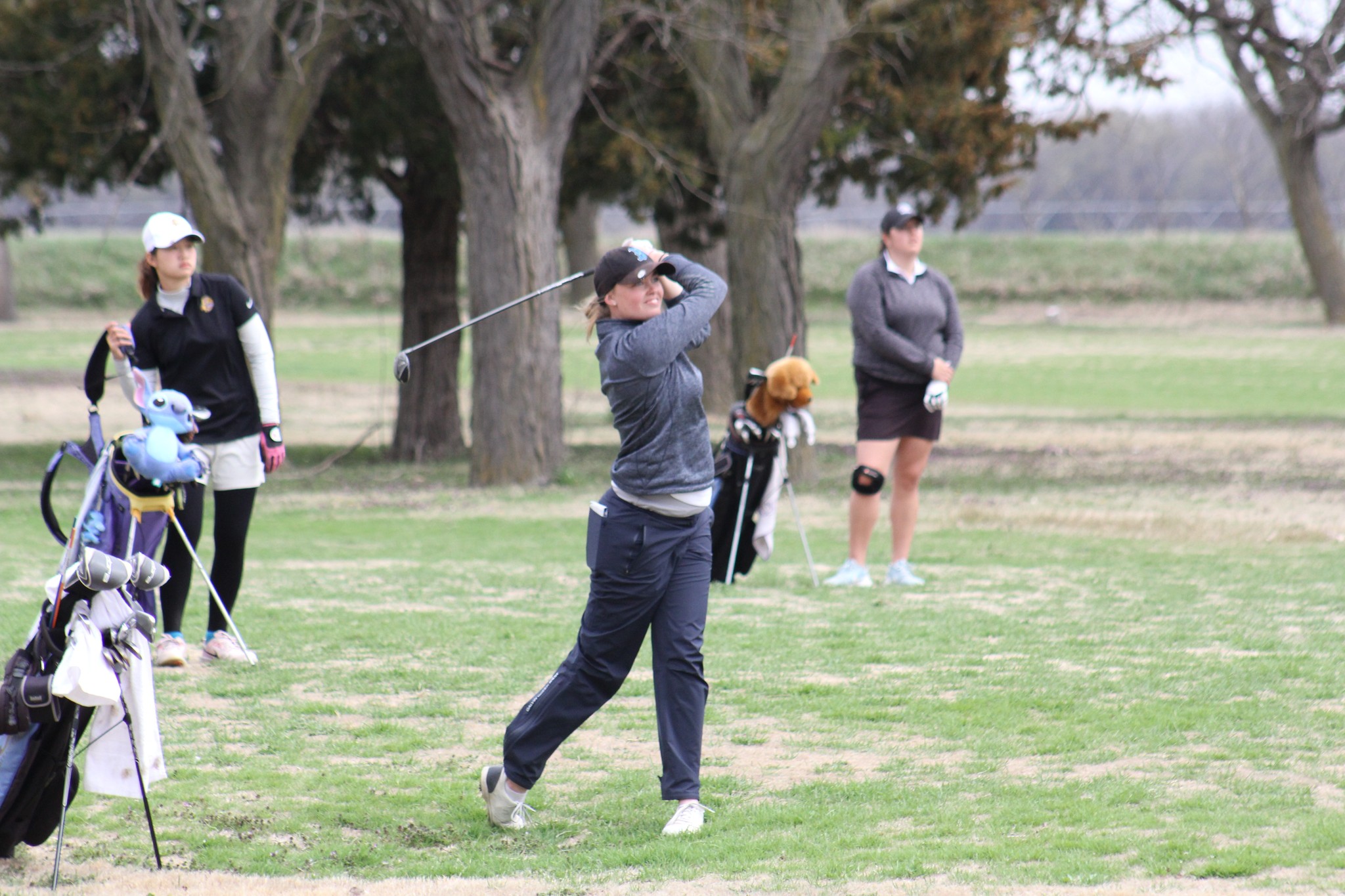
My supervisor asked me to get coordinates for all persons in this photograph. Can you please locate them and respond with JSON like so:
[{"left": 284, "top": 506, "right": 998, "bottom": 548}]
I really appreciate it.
[
  {"left": 822, "top": 202, "right": 963, "bottom": 589},
  {"left": 478, "top": 237, "right": 731, "bottom": 837},
  {"left": 104, "top": 212, "right": 287, "bottom": 665}
]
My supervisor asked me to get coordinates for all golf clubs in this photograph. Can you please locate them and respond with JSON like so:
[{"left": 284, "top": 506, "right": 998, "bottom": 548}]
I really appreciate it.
[
  {"left": 165, "top": 505, "right": 259, "bottom": 667},
  {"left": 732, "top": 411, "right": 817, "bottom": 450},
  {"left": 393, "top": 265, "right": 597, "bottom": 385},
  {"left": 783, "top": 469, "right": 824, "bottom": 589},
  {"left": 101, "top": 608, "right": 157, "bottom": 675}
]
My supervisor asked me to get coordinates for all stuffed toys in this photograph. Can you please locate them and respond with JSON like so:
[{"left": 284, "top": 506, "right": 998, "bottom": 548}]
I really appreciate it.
[
  {"left": 120, "top": 365, "right": 211, "bottom": 489},
  {"left": 747, "top": 357, "right": 821, "bottom": 427}
]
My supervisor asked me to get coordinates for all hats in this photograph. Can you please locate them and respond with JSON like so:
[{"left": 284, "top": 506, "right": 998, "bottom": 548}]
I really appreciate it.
[
  {"left": 141, "top": 211, "right": 204, "bottom": 253},
  {"left": 880, "top": 203, "right": 926, "bottom": 234},
  {"left": 593, "top": 246, "right": 676, "bottom": 299}
]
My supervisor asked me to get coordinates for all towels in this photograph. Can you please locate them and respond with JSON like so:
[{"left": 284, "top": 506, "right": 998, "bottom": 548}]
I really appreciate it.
[
  {"left": 45, "top": 560, "right": 168, "bottom": 801},
  {"left": 751, "top": 435, "right": 788, "bottom": 559}
]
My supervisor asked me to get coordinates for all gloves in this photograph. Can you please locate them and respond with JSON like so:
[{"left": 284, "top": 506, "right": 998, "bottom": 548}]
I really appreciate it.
[
  {"left": 259, "top": 423, "right": 285, "bottom": 473},
  {"left": 923, "top": 379, "right": 948, "bottom": 413}
]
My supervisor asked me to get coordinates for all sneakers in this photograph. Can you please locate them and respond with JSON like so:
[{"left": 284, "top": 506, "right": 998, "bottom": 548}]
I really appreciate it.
[
  {"left": 155, "top": 632, "right": 187, "bottom": 667},
  {"left": 883, "top": 560, "right": 925, "bottom": 587},
  {"left": 660, "top": 800, "right": 715, "bottom": 834},
  {"left": 822, "top": 558, "right": 873, "bottom": 587},
  {"left": 479, "top": 765, "right": 536, "bottom": 831},
  {"left": 202, "top": 629, "right": 258, "bottom": 666}
]
